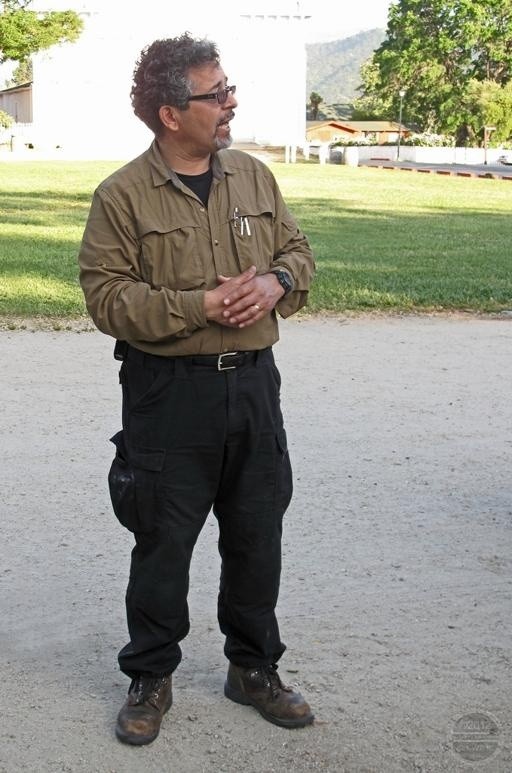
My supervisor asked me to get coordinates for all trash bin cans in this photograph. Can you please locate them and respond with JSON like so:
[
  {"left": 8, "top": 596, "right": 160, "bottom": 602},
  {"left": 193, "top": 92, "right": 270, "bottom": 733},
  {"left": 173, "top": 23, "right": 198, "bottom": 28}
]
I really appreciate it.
[{"left": 344, "top": 147, "right": 359, "bottom": 167}]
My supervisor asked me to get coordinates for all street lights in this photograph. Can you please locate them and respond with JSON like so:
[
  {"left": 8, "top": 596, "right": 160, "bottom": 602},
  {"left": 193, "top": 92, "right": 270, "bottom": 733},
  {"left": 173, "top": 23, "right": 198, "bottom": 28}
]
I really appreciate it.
[{"left": 397, "top": 90, "right": 405, "bottom": 160}]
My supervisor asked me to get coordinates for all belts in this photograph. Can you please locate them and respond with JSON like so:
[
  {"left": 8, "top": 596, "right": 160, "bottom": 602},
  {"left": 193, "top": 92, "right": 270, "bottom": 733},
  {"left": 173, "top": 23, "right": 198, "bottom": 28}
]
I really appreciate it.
[{"left": 121, "top": 342, "right": 248, "bottom": 371}]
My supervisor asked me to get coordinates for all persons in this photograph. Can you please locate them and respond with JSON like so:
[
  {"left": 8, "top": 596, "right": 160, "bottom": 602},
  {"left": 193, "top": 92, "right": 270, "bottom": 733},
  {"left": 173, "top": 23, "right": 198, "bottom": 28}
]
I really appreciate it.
[{"left": 78, "top": 31, "right": 316, "bottom": 746}]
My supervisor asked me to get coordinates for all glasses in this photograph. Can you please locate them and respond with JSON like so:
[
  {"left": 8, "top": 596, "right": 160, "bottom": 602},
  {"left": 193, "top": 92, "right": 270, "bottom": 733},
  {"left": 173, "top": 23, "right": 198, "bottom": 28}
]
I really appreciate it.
[{"left": 189, "top": 85, "right": 236, "bottom": 104}]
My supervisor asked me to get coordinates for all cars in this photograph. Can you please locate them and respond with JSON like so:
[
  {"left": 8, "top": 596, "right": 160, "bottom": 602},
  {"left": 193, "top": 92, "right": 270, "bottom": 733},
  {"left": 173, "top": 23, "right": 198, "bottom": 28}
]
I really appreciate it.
[{"left": 500, "top": 151, "right": 512, "bottom": 164}]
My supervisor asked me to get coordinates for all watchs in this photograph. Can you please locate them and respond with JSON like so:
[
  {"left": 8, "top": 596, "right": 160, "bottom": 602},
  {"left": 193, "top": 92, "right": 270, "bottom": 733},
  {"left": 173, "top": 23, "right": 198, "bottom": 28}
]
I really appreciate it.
[{"left": 267, "top": 271, "right": 293, "bottom": 294}]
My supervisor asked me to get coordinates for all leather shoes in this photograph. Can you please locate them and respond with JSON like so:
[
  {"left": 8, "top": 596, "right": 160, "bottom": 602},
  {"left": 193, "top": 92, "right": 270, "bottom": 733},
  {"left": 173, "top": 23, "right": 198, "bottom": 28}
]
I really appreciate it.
[
  {"left": 224, "top": 663, "right": 314, "bottom": 728},
  {"left": 115, "top": 676, "right": 172, "bottom": 747}
]
